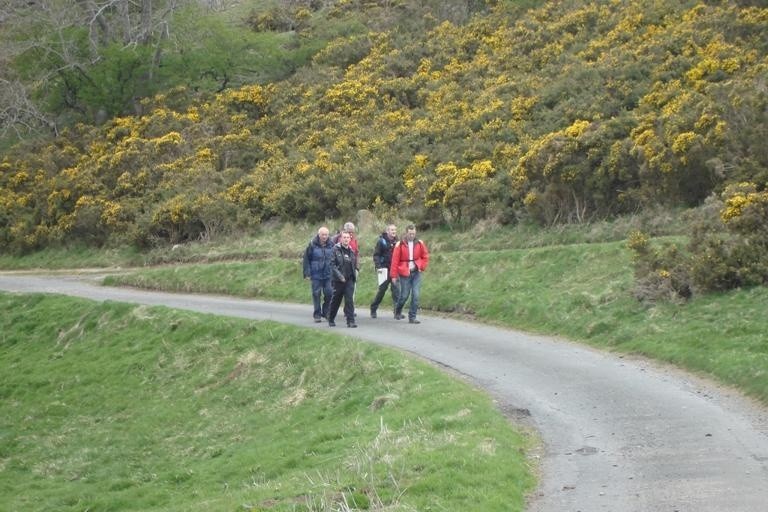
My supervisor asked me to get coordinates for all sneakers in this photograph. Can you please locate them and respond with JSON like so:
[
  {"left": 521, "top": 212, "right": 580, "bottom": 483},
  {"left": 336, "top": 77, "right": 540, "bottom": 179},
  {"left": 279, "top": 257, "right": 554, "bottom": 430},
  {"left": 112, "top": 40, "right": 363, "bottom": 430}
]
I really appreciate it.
[
  {"left": 392, "top": 311, "right": 406, "bottom": 321},
  {"left": 345, "top": 322, "right": 357, "bottom": 328},
  {"left": 312, "top": 313, "right": 329, "bottom": 324},
  {"left": 327, "top": 319, "right": 335, "bottom": 327},
  {"left": 369, "top": 305, "right": 377, "bottom": 319},
  {"left": 408, "top": 318, "right": 420, "bottom": 324}
]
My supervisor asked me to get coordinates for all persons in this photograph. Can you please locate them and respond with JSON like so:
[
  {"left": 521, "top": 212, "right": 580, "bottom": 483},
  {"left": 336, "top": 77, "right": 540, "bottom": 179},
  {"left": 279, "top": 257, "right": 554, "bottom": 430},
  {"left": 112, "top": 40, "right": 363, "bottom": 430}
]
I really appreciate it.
[
  {"left": 370, "top": 224, "right": 405, "bottom": 319},
  {"left": 302, "top": 222, "right": 360, "bottom": 328},
  {"left": 390, "top": 224, "right": 427, "bottom": 324}
]
